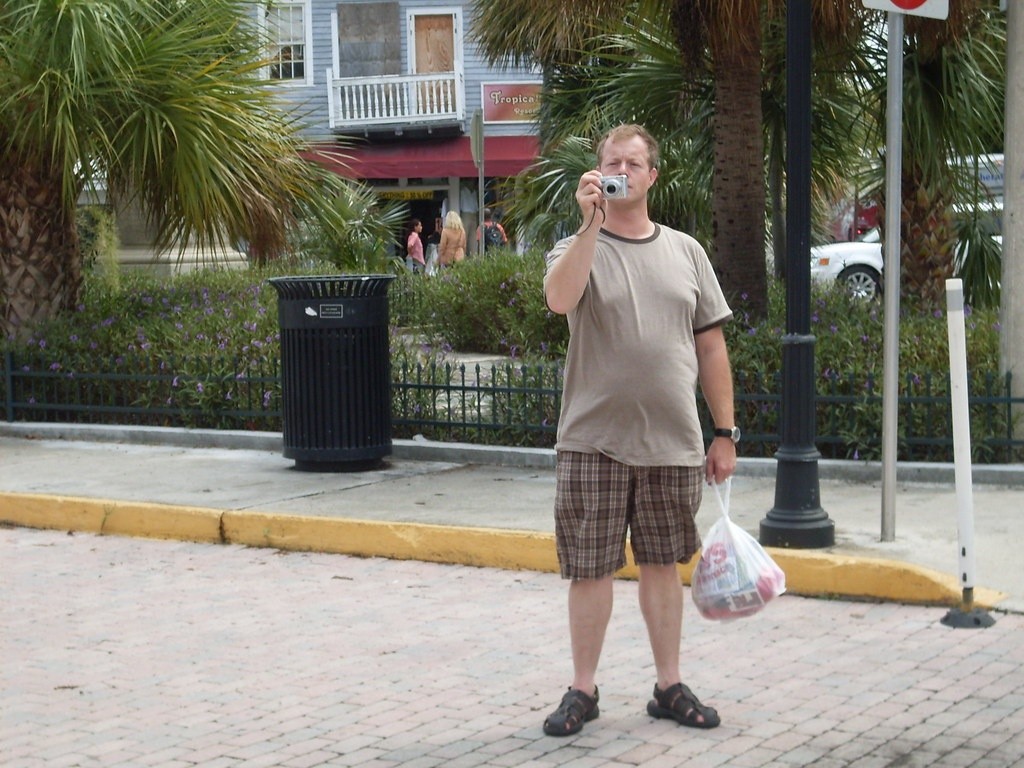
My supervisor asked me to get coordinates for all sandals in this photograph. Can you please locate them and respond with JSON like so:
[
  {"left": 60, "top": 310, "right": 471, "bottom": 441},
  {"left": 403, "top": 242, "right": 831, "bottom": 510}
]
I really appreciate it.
[
  {"left": 647, "top": 682, "right": 721, "bottom": 727},
  {"left": 542, "top": 684, "right": 601, "bottom": 736}
]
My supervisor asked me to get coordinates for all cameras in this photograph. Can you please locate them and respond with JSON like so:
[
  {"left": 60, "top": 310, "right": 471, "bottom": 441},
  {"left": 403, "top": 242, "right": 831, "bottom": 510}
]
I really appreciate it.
[{"left": 599, "top": 174, "right": 628, "bottom": 200}]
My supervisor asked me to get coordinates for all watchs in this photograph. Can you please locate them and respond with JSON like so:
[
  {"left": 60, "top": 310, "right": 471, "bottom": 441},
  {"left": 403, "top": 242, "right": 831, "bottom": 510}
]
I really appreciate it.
[{"left": 715, "top": 427, "right": 741, "bottom": 443}]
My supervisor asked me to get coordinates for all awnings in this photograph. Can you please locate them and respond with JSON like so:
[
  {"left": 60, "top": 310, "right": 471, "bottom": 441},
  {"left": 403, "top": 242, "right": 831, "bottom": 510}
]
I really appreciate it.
[{"left": 296, "top": 136, "right": 542, "bottom": 178}]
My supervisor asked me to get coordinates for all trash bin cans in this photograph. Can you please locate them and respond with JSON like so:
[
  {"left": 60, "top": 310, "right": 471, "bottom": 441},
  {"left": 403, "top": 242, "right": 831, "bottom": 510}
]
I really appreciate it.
[{"left": 264, "top": 274, "right": 397, "bottom": 475}]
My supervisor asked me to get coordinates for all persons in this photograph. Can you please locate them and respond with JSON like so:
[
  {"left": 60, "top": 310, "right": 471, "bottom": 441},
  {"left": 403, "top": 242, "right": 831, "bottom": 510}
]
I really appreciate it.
[
  {"left": 477, "top": 209, "right": 507, "bottom": 256},
  {"left": 408, "top": 220, "right": 426, "bottom": 274},
  {"left": 433, "top": 211, "right": 466, "bottom": 270},
  {"left": 544, "top": 126, "right": 741, "bottom": 737}
]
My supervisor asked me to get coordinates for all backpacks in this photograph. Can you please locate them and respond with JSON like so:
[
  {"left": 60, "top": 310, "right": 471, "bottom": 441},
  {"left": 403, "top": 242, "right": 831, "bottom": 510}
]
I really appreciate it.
[{"left": 484, "top": 221, "right": 506, "bottom": 252}]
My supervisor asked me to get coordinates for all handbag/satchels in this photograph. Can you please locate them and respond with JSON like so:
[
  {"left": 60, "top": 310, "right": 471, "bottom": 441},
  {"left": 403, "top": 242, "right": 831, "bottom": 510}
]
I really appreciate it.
[
  {"left": 690, "top": 474, "right": 788, "bottom": 621},
  {"left": 436, "top": 248, "right": 457, "bottom": 266}
]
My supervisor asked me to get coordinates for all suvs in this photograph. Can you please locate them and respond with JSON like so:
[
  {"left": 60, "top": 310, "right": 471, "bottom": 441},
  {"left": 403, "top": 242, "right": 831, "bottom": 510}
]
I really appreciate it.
[{"left": 809, "top": 203, "right": 1006, "bottom": 311}]
